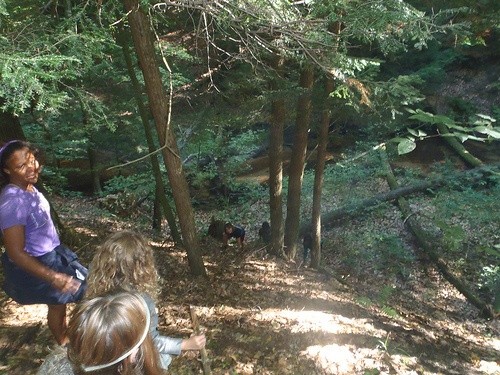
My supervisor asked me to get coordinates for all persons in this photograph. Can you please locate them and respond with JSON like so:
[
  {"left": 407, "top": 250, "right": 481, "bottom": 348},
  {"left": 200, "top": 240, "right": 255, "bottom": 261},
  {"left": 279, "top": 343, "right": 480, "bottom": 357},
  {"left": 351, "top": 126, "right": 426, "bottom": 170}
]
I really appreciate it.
[
  {"left": 0, "top": 140, "right": 90, "bottom": 348},
  {"left": 301, "top": 229, "right": 312, "bottom": 266},
  {"left": 37, "top": 292, "right": 164, "bottom": 375},
  {"left": 258, "top": 221, "right": 271, "bottom": 243},
  {"left": 220, "top": 222, "right": 246, "bottom": 250},
  {"left": 80, "top": 228, "right": 207, "bottom": 375}
]
